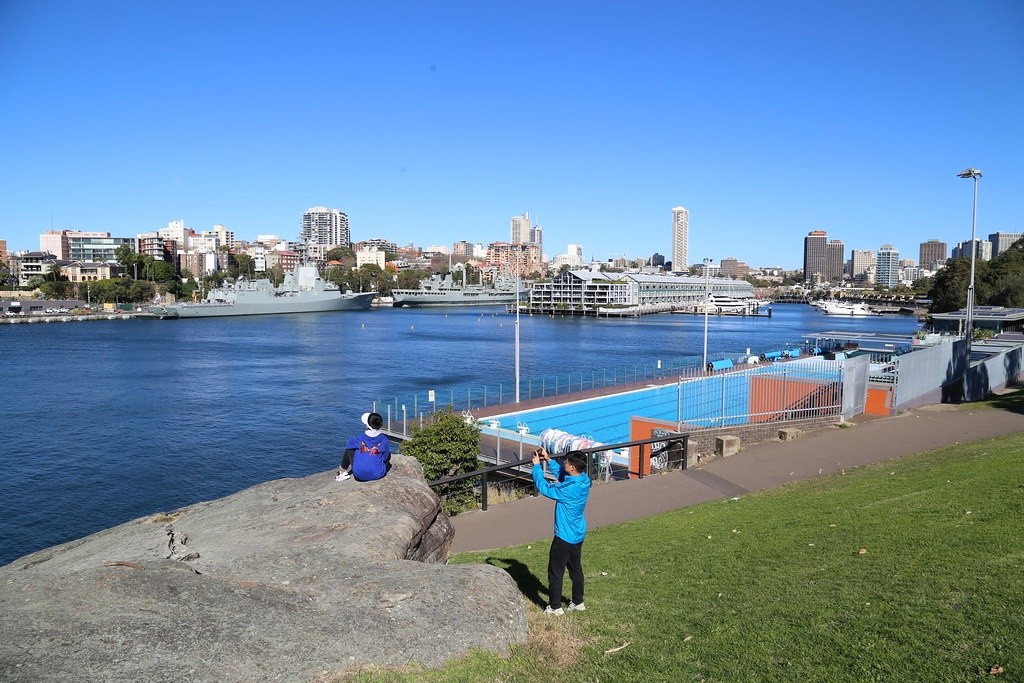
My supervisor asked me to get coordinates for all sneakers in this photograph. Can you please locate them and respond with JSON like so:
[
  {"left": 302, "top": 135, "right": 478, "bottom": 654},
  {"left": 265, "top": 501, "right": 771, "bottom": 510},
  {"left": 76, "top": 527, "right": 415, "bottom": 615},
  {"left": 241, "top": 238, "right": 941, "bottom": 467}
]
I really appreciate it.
[
  {"left": 543, "top": 605, "right": 564, "bottom": 616},
  {"left": 568, "top": 602, "right": 585, "bottom": 610},
  {"left": 335, "top": 465, "right": 350, "bottom": 482}
]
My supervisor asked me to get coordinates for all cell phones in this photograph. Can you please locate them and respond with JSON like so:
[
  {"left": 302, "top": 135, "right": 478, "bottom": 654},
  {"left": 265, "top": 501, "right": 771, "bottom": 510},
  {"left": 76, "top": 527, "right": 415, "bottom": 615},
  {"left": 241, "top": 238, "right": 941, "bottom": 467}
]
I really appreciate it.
[{"left": 534, "top": 449, "right": 542, "bottom": 458}]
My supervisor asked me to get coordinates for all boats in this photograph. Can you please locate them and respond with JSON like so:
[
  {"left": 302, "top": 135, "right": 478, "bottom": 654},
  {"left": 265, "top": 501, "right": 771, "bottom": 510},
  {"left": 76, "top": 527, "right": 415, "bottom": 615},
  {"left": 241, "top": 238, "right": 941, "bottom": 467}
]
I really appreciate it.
[
  {"left": 148, "top": 242, "right": 379, "bottom": 318},
  {"left": 821, "top": 301, "right": 873, "bottom": 315},
  {"left": 391, "top": 256, "right": 529, "bottom": 307}
]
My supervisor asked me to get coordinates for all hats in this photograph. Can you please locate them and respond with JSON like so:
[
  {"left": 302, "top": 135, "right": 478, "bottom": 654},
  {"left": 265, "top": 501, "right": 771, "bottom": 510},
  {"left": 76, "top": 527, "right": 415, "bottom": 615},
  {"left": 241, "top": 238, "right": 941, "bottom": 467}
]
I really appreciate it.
[{"left": 361, "top": 412, "right": 383, "bottom": 431}]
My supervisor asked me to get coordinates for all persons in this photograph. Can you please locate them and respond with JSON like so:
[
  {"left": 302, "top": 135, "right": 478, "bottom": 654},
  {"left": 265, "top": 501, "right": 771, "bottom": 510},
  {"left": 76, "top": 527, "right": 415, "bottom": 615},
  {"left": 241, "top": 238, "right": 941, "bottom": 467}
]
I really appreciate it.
[
  {"left": 532, "top": 446, "right": 590, "bottom": 616},
  {"left": 335, "top": 413, "right": 391, "bottom": 482}
]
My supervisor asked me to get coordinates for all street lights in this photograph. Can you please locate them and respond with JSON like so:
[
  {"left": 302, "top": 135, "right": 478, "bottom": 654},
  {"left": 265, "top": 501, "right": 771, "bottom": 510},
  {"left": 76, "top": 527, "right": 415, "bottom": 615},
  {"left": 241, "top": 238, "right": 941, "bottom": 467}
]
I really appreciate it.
[
  {"left": 511, "top": 243, "right": 527, "bottom": 404},
  {"left": 703, "top": 258, "right": 713, "bottom": 372},
  {"left": 957, "top": 168, "right": 982, "bottom": 401}
]
